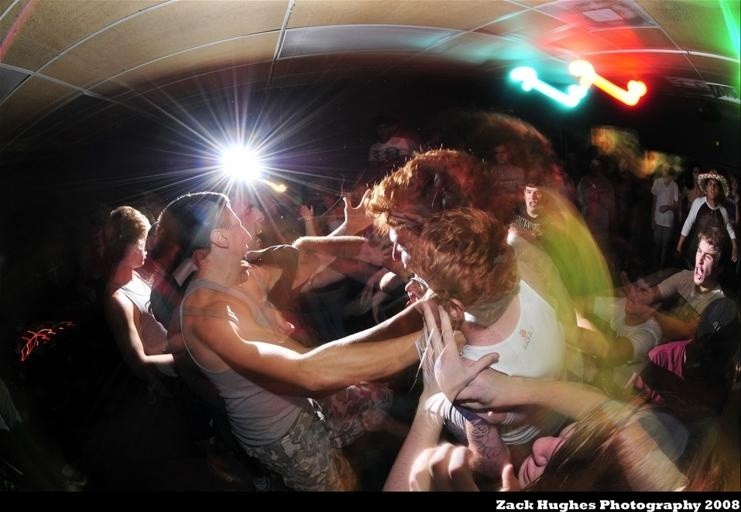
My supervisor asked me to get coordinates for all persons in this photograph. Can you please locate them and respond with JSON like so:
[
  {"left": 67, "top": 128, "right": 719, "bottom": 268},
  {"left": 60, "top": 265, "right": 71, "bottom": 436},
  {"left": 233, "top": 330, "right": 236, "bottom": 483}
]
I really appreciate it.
[{"left": 0, "top": 108, "right": 741, "bottom": 493}]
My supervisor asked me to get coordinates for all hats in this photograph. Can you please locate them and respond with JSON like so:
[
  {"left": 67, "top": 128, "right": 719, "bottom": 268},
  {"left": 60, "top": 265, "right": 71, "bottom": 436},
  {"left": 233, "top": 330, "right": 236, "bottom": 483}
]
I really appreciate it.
[{"left": 698, "top": 170, "right": 730, "bottom": 198}]
